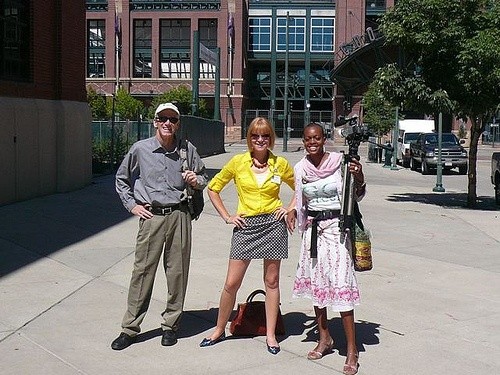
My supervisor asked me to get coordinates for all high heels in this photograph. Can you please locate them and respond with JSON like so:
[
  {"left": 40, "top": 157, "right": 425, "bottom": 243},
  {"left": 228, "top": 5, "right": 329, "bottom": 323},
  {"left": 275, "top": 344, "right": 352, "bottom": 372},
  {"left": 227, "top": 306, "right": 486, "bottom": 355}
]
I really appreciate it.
[
  {"left": 307, "top": 336, "right": 334, "bottom": 360},
  {"left": 342, "top": 350, "right": 359, "bottom": 375}
]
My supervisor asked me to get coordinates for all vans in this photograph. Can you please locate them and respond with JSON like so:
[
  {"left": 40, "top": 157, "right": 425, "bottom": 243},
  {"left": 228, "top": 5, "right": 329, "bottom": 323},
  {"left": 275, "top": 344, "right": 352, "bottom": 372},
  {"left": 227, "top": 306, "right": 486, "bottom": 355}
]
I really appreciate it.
[{"left": 396, "top": 118, "right": 435, "bottom": 168}]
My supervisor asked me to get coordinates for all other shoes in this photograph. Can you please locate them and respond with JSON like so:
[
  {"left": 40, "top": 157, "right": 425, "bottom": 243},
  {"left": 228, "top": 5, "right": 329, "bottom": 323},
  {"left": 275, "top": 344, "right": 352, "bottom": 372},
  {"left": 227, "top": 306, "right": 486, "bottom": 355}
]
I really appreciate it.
[
  {"left": 160, "top": 330, "right": 177, "bottom": 346},
  {"left": 199, "top": 331, "right": 225, "bottom": 347},
  {"left": 111, "top": 332, "right": 138, "bottom": 350},
  {"left": 266, "top": 339, "right": 280, "bottom": 355}
]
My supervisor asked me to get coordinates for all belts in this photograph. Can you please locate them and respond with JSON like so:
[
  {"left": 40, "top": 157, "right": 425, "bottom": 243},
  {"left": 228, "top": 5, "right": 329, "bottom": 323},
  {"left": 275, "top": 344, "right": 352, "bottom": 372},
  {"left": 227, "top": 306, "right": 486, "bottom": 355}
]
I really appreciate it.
[
  {"left": 146, "top": 201, "right": 184, "bottom": 216},
  {"left": 303, "top": 209, "right": 341, "bottom": 258}
]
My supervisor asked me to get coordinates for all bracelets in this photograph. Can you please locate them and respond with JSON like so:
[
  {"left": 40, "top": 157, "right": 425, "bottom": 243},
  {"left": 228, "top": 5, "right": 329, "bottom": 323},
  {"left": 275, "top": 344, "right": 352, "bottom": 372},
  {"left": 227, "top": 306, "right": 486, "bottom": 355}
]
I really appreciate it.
[{"left": 226, "top": 215, "right": 232, "bottom": 224}]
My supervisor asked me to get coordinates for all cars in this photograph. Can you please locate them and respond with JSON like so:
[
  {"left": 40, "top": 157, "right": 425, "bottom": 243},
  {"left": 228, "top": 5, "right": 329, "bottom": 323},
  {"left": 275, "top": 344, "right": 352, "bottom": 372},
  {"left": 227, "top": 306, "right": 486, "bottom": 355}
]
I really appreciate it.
[
  {"left": 491, "top": 152, "right": 500, "bottom": 210},
  {"left": 410, "top": 133, "right": 468, "bottom": 175}
]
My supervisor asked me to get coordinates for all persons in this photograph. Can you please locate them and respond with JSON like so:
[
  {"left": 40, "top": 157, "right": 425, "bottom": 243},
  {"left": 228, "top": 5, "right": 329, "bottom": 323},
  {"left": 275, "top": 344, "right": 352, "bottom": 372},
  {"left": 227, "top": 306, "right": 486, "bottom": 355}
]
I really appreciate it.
[
  {"left": 205, "top": 116, "right": 298, "bottom": 353},
  {"left": 286, "top": 122, "right": 367, "bottom": 375},
  {"left": 110, "top": 102, "right": 209, "bottom": 349}
]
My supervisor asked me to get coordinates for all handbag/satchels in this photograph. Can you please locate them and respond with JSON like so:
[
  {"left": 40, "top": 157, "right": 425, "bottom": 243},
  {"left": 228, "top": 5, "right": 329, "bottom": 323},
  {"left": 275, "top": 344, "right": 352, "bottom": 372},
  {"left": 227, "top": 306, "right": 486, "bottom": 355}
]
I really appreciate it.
[
  {"left": 229, "top": 290, "right": 285, "bottom": 336},
  {"left": 353, "top": 221, "right": 373, "bottom": 272}
]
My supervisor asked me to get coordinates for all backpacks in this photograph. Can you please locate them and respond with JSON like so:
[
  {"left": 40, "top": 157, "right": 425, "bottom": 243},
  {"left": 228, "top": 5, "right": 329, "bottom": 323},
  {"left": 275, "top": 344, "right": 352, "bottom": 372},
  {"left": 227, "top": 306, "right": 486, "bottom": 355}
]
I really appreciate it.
[{"left": 180, "top": 139, "right": 205, "bottom": 221}]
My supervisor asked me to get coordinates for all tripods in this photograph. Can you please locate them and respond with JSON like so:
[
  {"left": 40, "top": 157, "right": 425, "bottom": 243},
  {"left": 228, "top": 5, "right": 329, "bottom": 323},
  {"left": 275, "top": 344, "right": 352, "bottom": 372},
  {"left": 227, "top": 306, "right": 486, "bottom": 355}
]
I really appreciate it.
[{"left": 339, "top": 133, "right": 394, "bottom": 233}]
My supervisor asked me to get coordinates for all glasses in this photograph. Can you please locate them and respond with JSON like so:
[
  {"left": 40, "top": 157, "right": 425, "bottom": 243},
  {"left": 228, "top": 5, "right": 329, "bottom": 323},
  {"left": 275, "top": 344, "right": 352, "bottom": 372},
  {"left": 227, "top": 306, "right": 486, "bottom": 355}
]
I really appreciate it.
[
  {"left": 153, "top": 117, "right": 178, "bottom": 124},
  {"left": 250, "top": 134, "right": 270, "bottom": 140}
]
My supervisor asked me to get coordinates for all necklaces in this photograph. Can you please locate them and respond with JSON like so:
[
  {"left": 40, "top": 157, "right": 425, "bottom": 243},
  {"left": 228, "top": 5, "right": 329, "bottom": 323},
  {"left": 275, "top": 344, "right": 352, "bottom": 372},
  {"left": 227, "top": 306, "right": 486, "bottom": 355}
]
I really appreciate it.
[{"left": 252, "top": 152, "right": 268, "bottom": 169}]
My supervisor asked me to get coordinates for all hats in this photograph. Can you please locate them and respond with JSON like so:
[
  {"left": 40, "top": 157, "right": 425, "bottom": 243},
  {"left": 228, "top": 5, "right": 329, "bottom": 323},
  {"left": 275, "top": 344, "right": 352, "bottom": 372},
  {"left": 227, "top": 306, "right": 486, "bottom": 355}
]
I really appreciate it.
[{"left": 155, "top": 102, "right": 180, "bottom": 116}]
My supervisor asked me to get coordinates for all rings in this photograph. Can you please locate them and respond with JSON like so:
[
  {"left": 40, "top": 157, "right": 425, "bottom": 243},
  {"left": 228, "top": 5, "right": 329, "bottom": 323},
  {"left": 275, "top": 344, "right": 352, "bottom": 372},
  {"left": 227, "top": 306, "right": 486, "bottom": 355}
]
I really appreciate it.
[{"left": 354, "top": 165, "right": 358, "bottom": 170}]
